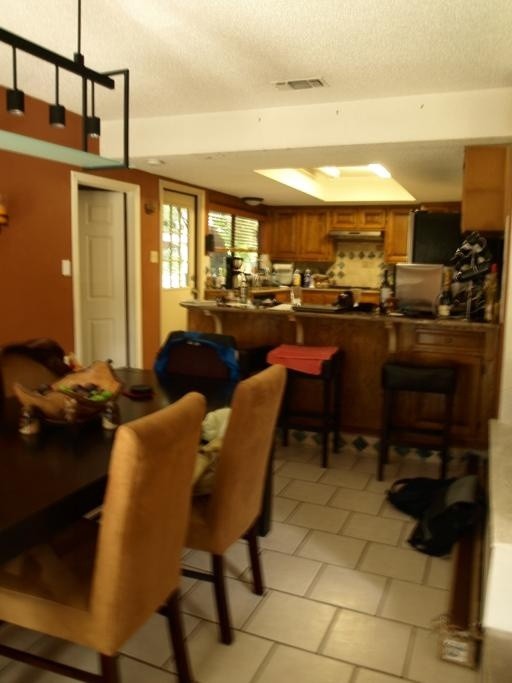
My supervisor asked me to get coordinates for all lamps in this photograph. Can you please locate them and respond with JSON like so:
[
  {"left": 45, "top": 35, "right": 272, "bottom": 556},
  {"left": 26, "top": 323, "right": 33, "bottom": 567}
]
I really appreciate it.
[{"left": 4, "top": 44, "right": 102, "bottom": 139}]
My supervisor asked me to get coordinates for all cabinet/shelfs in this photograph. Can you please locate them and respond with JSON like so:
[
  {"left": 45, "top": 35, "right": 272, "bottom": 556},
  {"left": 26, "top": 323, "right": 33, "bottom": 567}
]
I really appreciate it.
[
  {"left": 333, "top": 206, "right": 383, "bottom": 232},
  {"left": 384, "top": 207, "right": 411, "bottom": 263},
  {"left": 268, "top": 208, "right": 330, "bottom": 261}
]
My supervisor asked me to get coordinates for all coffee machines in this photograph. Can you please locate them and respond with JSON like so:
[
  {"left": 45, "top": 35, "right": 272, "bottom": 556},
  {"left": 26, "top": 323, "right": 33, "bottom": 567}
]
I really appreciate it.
[{"left": 225, "top": 257, "right": 247, "bottom": 290}]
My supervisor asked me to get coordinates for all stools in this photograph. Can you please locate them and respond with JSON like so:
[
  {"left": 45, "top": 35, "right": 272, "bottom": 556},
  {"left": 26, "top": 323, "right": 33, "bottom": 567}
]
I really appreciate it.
[{"left": 266, "top": 343, "right": 458, "bottom": 486}]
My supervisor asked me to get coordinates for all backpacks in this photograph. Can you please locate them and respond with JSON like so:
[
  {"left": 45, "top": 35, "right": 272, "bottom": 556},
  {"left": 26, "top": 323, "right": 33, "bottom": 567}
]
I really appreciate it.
[{"left": 383, "top": 474, "right": 479, "bottom": 557}]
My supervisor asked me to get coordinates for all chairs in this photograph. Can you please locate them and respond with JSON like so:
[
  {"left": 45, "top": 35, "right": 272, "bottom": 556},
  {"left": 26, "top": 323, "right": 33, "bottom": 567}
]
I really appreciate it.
[
  {"left": 181, "top": 363, "right": 288, "bottom": 647},
  {"left": 1, "top": 392, "right": 208, "bottom": 683}
]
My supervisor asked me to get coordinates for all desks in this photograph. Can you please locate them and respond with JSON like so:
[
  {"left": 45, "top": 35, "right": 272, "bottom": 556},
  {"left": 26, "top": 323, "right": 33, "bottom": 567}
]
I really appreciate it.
[{"left": 0, "top": 367, "right": 177, "bottom": 546}]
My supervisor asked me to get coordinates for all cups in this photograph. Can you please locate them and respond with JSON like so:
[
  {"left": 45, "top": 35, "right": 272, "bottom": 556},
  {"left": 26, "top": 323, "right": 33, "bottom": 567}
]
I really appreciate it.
[{"left": 438, "top": 305, "right": 450, "bottom": 317}]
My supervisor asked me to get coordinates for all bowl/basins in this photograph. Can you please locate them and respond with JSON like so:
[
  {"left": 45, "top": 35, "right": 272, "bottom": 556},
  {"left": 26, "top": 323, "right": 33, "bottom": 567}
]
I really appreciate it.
[{"left": 14, "top": 356, "right": 121, "bottom": 425}]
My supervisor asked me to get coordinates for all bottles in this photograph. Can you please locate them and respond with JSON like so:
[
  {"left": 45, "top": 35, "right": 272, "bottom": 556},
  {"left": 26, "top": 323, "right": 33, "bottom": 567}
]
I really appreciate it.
[
  {"left": 239, "top": 280, "right": 249, "bottom": 303},
  {"left": 293, "top": 267, "right": 330, "bottom": 289},
  {"left": 16, "top": 406, "right": 43, "bottom": 437},
  {"left": 103, "top": 401, "right": 122, "bottom": 430},
  {"left": 380, "top": 270, "right": 393, "bottom": 314}
]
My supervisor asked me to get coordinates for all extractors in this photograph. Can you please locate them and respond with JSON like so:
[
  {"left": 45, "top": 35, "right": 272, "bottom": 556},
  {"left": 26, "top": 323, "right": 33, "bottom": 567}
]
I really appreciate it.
[{"left": 330, "top": 229, "right": 385, "bottom": 241}]
